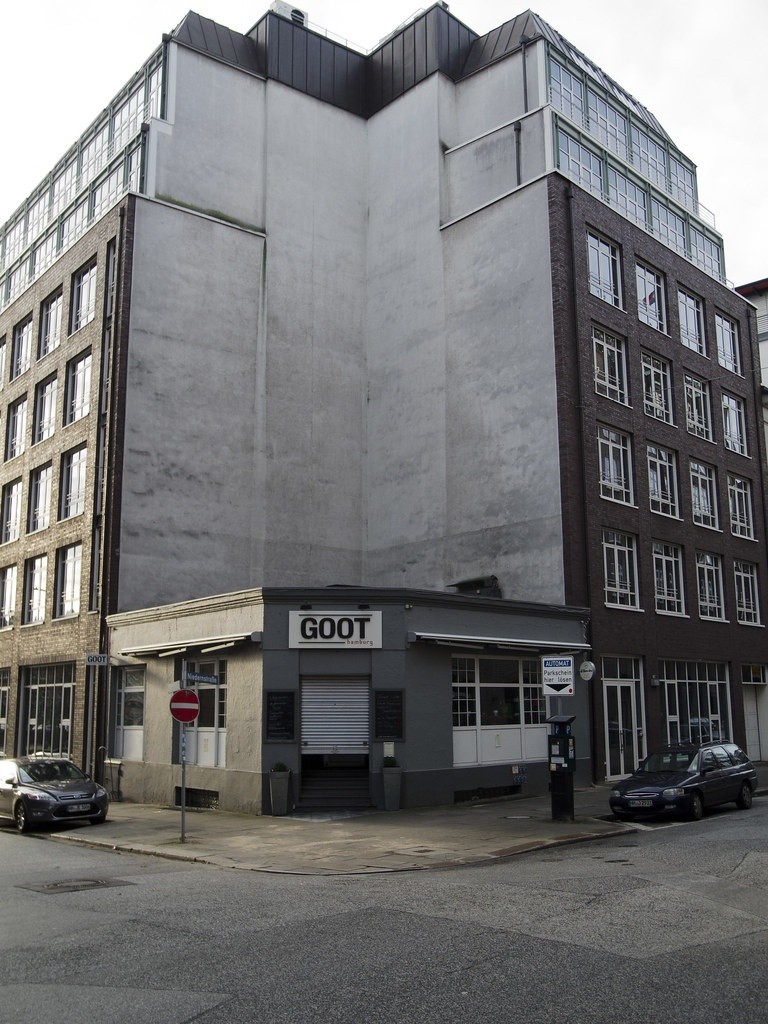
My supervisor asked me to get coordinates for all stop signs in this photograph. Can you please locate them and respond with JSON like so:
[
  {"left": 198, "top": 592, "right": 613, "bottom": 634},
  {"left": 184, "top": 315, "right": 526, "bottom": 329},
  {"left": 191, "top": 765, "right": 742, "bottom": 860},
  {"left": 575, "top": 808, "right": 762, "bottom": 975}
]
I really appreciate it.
[{"left": 169, "top": 688, "right": 200, "bottom": 724}]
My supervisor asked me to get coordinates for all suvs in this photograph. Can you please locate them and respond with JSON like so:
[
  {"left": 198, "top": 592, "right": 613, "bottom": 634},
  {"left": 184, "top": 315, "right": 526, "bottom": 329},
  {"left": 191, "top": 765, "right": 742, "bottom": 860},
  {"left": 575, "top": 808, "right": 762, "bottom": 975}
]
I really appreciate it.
[{"left": 607, "top": 738, "right": 758, "bottom": 823}]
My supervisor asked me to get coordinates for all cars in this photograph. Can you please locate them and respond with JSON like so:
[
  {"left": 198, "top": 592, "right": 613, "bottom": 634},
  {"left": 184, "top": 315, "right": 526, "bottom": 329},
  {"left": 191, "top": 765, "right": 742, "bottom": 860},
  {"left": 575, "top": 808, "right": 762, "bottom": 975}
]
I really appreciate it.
[{"left": 0, "top": 757, "right": 109, "bottom": 835}]
